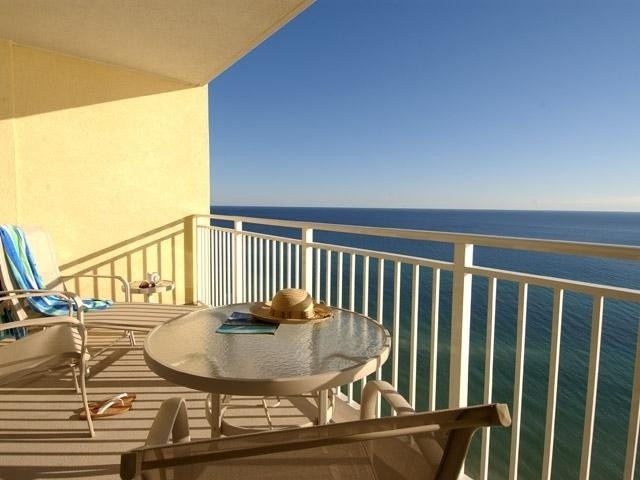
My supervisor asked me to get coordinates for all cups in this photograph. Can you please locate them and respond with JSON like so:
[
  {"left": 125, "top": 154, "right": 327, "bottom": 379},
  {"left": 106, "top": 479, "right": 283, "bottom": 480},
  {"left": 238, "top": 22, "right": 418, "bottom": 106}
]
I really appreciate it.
[{"left": 147, "top": 271, "right": 160, "bottom": 285}]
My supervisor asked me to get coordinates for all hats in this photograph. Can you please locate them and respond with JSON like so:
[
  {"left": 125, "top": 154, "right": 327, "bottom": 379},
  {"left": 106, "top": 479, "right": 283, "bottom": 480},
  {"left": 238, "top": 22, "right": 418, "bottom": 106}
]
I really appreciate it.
[{"left": 249, "top": 288, "right": 332, "bottom": 323}]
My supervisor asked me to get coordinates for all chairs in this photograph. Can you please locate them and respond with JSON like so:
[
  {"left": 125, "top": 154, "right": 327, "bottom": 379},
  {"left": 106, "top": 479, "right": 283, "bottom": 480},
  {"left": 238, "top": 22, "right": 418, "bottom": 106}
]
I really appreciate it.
[
  {"left": 120, "top": 379, "right": 513, "bottom": 480},
  {"left": 0, "top": 225, "right": 136, "bottom": 440}
]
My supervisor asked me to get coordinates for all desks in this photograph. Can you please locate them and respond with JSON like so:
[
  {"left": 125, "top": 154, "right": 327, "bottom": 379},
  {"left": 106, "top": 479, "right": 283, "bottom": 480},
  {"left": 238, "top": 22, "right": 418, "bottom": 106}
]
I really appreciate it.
[
  {"left": 120, "top": 280, "right": 173, "bottom": 304},
  {"left": 142, "top": 299, "right": 393, "bottom": 441}
]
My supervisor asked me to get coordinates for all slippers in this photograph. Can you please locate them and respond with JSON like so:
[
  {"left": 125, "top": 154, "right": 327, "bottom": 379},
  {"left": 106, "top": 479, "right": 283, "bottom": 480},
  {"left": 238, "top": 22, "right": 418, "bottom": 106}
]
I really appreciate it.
[{"left": 80, "top": 393, "right": 136, "bottom": 419}]
